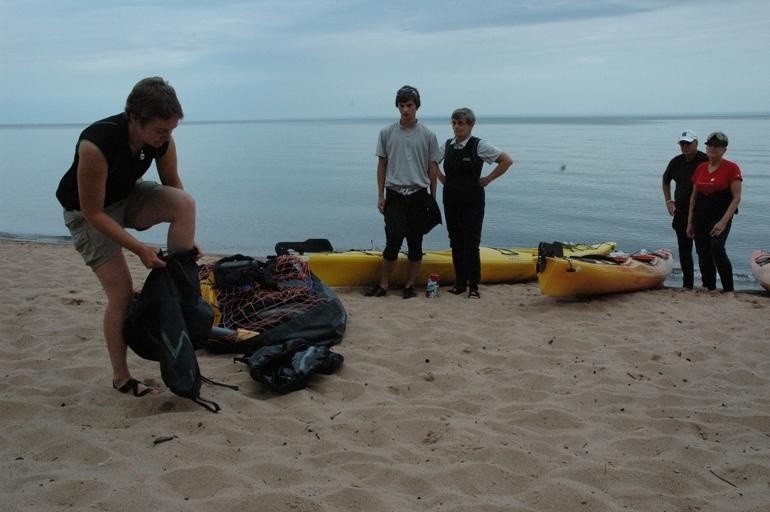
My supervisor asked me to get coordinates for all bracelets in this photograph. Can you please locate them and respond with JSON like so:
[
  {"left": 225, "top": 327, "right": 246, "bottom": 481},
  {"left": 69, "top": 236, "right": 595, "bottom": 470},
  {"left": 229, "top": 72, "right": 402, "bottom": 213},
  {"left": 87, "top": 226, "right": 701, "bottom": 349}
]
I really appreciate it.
[{"left": 665, "top": 199, "right": 674, "bottom": 206}]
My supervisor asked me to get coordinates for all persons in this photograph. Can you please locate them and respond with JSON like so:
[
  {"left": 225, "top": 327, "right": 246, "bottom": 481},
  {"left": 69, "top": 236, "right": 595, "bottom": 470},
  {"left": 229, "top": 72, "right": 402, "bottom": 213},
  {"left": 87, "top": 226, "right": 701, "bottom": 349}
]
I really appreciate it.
[
  {"left": 435, "top": 107, "right": 513, "bottom": 299},
  {"left": 686, "top": 131, "right": 742, "bottom": 296},
  {"left": 661, "top": 129, "right": 710, "bottom": 292},
  {"left": 365, "top": 84, "right": 442, "bottom": 298},
  {"left": 55, "top": 76, "right": 205, "bottom": 397}
]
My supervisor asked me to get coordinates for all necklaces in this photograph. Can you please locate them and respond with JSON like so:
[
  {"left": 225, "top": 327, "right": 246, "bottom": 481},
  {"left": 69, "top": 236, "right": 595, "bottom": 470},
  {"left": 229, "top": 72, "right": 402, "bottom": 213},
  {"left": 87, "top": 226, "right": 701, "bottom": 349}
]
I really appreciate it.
[{"left": 128, "top": 133, "right": 148, "bottom": 160}]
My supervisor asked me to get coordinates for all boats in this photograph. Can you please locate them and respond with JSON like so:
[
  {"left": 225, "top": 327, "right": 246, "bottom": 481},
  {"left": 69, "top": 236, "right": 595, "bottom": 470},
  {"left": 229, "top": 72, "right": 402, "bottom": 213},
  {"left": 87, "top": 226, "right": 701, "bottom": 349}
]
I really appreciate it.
[
  {"left": 274, "top": 237, "right": 616, "bottom": 290},
  {"left": 536, "top": 247, "right": 674, "bottom": 298},
  {"left": 751, "top": 248, "right": 769, "bottom": 292}
]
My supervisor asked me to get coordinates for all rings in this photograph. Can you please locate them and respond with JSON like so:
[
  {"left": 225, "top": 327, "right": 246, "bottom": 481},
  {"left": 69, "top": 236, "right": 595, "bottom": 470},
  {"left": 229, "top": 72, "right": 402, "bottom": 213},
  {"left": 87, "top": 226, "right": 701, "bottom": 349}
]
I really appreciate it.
[{"left": 717, "top": 230, "right": 720, "bottom": 232}]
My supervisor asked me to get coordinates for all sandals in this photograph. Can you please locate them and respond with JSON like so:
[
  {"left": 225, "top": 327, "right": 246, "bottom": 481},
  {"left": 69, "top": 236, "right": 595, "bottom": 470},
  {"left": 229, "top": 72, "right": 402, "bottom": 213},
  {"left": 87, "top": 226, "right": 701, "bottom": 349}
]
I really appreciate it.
[{"left": 112, "top": 379, "right": 160, "bottom": 397}]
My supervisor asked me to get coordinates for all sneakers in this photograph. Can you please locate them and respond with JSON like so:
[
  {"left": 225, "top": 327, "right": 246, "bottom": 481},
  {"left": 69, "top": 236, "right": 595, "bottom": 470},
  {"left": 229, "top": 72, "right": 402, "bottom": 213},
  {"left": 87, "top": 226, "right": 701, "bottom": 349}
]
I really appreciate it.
[
  {"left": 403, "top": 287, "right": 412, "bottom": 299},
  {"left": 365, "top": 287, "right": 387, "bottom": 297}
]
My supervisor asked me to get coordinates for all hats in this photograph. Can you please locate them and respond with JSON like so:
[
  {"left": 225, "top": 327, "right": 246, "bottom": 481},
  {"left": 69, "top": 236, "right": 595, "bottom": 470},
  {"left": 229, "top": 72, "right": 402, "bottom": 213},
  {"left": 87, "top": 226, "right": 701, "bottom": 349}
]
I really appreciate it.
[
  {"left": 676, "top": 130, "right": 697, "bottom": 143},
  {"left": 704, "top": 131, "right": 728, "bottom": 146}
]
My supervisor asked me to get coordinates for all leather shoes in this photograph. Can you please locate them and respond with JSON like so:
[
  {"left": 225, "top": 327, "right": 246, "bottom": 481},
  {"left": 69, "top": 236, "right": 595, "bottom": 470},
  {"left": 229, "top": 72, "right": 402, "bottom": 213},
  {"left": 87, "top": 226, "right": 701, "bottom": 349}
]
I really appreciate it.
[
  {"left": 470, "top": 286, "right": 479, "bottom": 296},
  {"left": 448, "top": 286, "right": 466, "bottom": 295}
]
